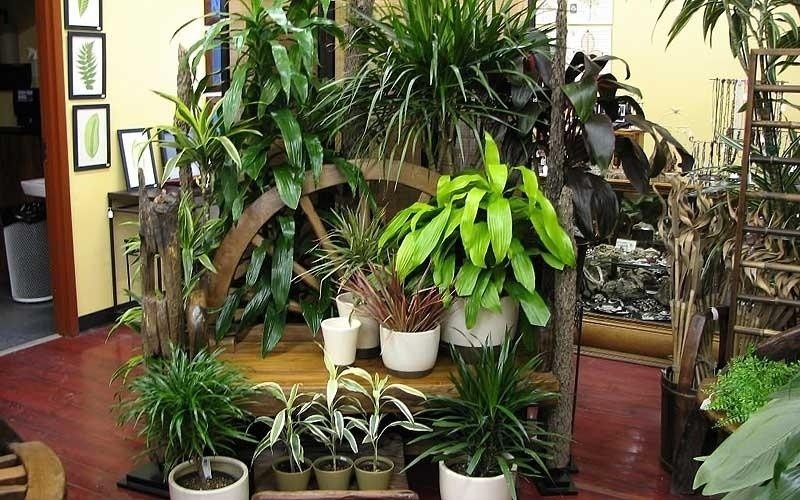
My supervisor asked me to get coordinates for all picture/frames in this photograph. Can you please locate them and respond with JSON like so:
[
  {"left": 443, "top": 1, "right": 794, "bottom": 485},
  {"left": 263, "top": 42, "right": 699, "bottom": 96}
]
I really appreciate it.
[
  {"left": 114, "top": 125, "right": 200, "bottom": 189},
  {"left": 62, "top": 0, "right": 111, "bottom": 171}
]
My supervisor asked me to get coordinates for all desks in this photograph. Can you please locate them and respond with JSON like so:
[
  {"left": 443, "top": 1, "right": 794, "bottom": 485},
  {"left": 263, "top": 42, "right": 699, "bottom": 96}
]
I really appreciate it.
[{"left": 108, "top": 180, "right": 207, "bottom": 325}]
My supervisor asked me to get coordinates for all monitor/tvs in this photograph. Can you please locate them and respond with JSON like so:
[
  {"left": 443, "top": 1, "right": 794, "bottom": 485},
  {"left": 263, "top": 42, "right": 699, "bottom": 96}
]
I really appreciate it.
[{"left": 16, "top": 89, "right": 34, "bottom": 104}]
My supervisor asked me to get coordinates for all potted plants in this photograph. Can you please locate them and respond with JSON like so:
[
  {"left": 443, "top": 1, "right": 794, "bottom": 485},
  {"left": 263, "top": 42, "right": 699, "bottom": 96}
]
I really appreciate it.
[
  {"left": 108, "top": 339, "right": 264, "bottom": 500},
  {"left": 304, "top": 340, "right": 374, "bottom": 491},
  {"left": 246, "top": 382, "right": 329, "bottom": 493},
  {"left": 320, "top": 131, "right": 574, "bottom": 378},
  {"left": 400, "top": 335, "right": 577, "bottom": 500},
  {"left": 338, "top": 367, "right": 434, "bottom": 493}
]
